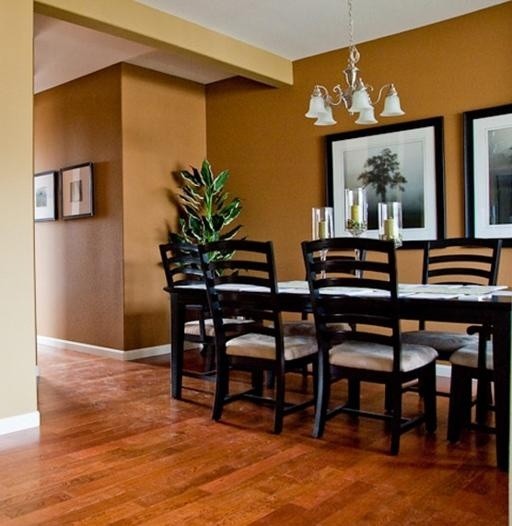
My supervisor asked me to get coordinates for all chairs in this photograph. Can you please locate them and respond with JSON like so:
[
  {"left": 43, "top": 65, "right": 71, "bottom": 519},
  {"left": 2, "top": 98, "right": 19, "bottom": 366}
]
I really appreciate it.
[
  {"left": 285, "top": 237, "right": 368, "bottom": 376},
  {"left": 300, "top": 237, "right": 440, "bottom": 456},
  {"left": 446, "top": 325, "right": 497, "bottom": 444},
  {"left": 157, "top": 242, "right": 261, "bottom": 396},
  {"left": 198, "top": 238, "right": 320, "bottom": 435},
  {"left": 382, "top": 236, "right": 504, "bottom": 422}
]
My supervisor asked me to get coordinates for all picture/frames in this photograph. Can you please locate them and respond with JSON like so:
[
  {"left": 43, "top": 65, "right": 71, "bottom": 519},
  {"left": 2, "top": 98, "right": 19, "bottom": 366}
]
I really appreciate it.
[
  {"left": 59, "top": 161, "right": 95, "bottom": 220},
  {"left": 323, "top": 114, "right": 446, "bottom": 249},
  {"left": 33, "top": 170, "right": 59, "bottom": 223},
  {"left": 462, "top": 102, "right": 512, "bottom": 249}
]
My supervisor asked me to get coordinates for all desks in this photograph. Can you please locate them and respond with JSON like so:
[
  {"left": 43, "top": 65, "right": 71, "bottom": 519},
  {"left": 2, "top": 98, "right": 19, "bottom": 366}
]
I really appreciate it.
[{"left": 159, "top": 278, "right": 511, "bottom": 475}]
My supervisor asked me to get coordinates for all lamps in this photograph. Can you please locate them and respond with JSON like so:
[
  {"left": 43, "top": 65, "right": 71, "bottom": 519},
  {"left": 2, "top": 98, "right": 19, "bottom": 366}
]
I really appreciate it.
[{"left": 301, "top": 0, "right": 407, "bottom": 130}]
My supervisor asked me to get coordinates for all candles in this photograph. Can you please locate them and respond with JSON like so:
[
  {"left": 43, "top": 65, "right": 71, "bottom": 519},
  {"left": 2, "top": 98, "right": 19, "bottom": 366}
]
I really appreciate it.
[
  {"left": 317, "top": 218, "right": 328, "bottom": 241},
  {"left": 383, "top": 214, "right": 397, "bottom": 240},
  {"left": 350, "top": 200, "right": 362, "bottom": 229}
]
